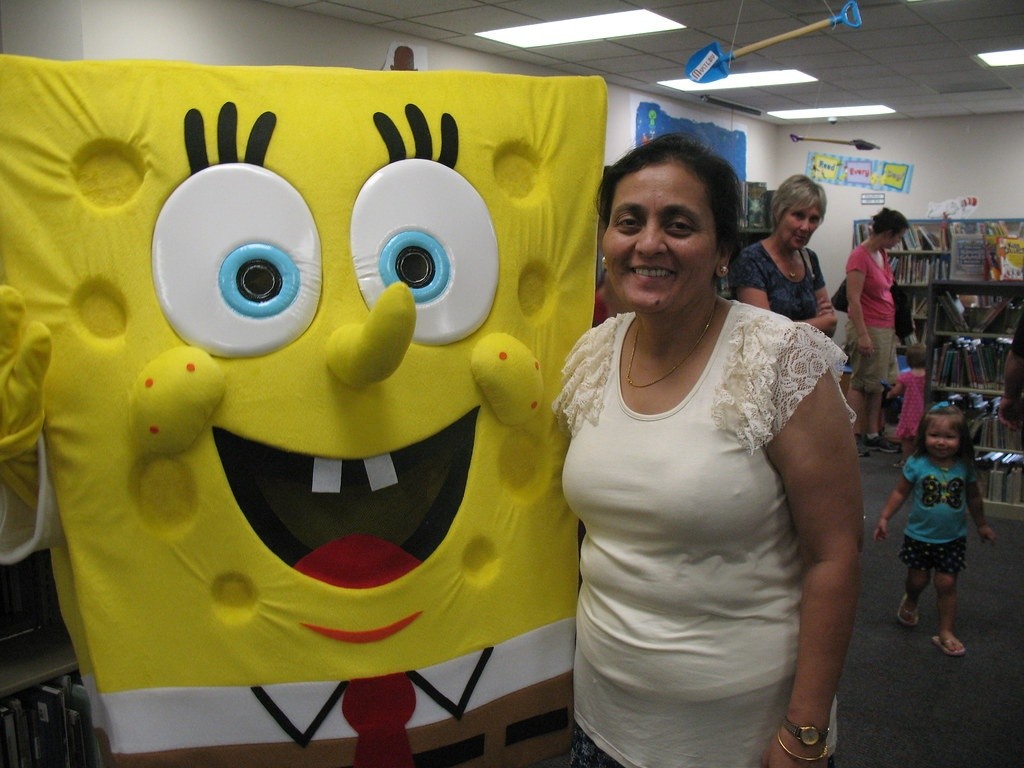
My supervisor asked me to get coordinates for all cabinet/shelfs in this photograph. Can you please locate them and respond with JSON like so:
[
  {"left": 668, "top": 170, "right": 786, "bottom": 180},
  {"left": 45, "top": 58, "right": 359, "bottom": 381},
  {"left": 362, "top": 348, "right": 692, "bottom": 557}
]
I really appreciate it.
[
  {"left": 853, "top": 219, "right": 1024, "bottom": 521},
  {"left": 717, "top": 224, "right": 771, "bottom": 297}
]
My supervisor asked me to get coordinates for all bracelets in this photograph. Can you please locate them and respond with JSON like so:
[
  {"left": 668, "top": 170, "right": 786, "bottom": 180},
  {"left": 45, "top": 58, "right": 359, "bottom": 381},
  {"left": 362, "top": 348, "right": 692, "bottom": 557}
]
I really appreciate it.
[{"left": 777, "top": 725, "right": 829, "bottom": 762}]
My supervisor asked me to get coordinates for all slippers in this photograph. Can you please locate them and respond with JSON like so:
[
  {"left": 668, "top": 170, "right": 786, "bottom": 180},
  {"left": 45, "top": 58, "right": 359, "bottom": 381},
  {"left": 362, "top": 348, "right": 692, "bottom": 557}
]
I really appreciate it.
[
  {"left": 931, "top": 633, "right": 966, "bottom": 657},
  {"left": 897, "top": 592, "right": 922, "bottom": 625}
]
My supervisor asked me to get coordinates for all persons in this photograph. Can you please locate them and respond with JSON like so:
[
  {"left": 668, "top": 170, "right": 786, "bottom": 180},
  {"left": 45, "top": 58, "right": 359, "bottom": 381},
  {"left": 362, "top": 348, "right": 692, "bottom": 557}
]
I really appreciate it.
[
  {"left": 556, "top": 130, "right": 864, "bottom": 768},
  {"left": 870, "top": 398, "right": 996, "bottom": 656},
  {"left": 846, "top": 208, "right": 910, "bottom": 453},
  {"left": 886, "top": 341, "right": 928, "bottom": 468},
  {"left": 997, "top": 314, "right": 1024, "bottom": 433},
  {"left": 733, "top": 175, "right": 836, "bottom": 344},
  {"left": 593, "top": 268, "right": 609, "bottom": 326}
]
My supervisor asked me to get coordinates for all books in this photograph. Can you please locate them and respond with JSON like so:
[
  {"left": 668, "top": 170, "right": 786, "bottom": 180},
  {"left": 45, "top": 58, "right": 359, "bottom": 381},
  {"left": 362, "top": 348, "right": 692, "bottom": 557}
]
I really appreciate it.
[
  {"left": 0, "top": 551, "right": 93, "bottom": 767},
  {"left": 718, "top": 181, "right": 776, "bottom": 297},
  {"left": 856, "top": 222, "right": 1024, "bottom": 504}
]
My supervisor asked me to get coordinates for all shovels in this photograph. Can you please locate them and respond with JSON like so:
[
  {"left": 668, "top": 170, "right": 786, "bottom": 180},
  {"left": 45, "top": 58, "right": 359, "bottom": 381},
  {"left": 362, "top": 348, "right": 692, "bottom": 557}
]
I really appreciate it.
[
  {"left": 790, "top": 133, "right": 880, "bottom": 150},
  {"left": 685, "top": 0, "right": 863, "bottom": 83}
]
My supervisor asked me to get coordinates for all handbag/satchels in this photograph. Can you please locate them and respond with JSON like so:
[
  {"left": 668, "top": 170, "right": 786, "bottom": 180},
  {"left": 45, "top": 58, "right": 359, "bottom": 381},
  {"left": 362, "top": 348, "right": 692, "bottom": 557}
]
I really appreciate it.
[
  {"left": 890, "top": 280, "right": 913, "bottom": 337},
  {"left": 831, "top": 279, "right": 849, "bottom": 313}
]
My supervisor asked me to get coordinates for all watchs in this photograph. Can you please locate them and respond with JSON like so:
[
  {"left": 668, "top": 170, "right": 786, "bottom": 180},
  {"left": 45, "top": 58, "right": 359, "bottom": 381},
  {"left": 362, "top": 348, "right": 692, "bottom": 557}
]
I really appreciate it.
[{"left": 782, "top": 716, "right": 830, "bottom": 746}]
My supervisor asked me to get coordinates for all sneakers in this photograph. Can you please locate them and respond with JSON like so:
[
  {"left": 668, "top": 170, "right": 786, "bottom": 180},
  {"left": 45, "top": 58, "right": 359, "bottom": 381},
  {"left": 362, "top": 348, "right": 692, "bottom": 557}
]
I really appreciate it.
[
  {"left": 855, "top": 442, "right": 869, "bottom": 457},
  {"left": 862, "top": 434, "right": 900, "bottom": 452}
]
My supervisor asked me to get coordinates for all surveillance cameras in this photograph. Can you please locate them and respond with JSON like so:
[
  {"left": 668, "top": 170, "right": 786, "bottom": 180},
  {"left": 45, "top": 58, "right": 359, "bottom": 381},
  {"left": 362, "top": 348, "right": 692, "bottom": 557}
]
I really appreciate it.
[{"left": 827, "top": 117, "right": 838, "bottom": 125}]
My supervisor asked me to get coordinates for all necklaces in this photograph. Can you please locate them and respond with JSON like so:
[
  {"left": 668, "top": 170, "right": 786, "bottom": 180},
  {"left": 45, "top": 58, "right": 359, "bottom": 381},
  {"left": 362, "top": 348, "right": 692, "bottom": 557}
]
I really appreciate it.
[
  {"left": 779, "top": 251, "right": 796, "bottom": 278},
  {"left": 625, "top": 299, "right": 718, "bottom": 387}
]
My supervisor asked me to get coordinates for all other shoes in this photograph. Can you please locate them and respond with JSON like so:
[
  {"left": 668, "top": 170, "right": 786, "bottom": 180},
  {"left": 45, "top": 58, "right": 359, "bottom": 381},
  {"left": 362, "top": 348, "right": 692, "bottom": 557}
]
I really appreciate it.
[{"left": 893, "top": 458, "right": 907, "bottom": 468}]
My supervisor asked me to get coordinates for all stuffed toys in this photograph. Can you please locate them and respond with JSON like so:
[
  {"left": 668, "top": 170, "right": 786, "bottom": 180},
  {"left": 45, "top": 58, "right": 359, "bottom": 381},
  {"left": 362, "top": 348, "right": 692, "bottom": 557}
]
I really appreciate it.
[{"left": 0, "top": 45, "right": 609, "bottom": 768}]
